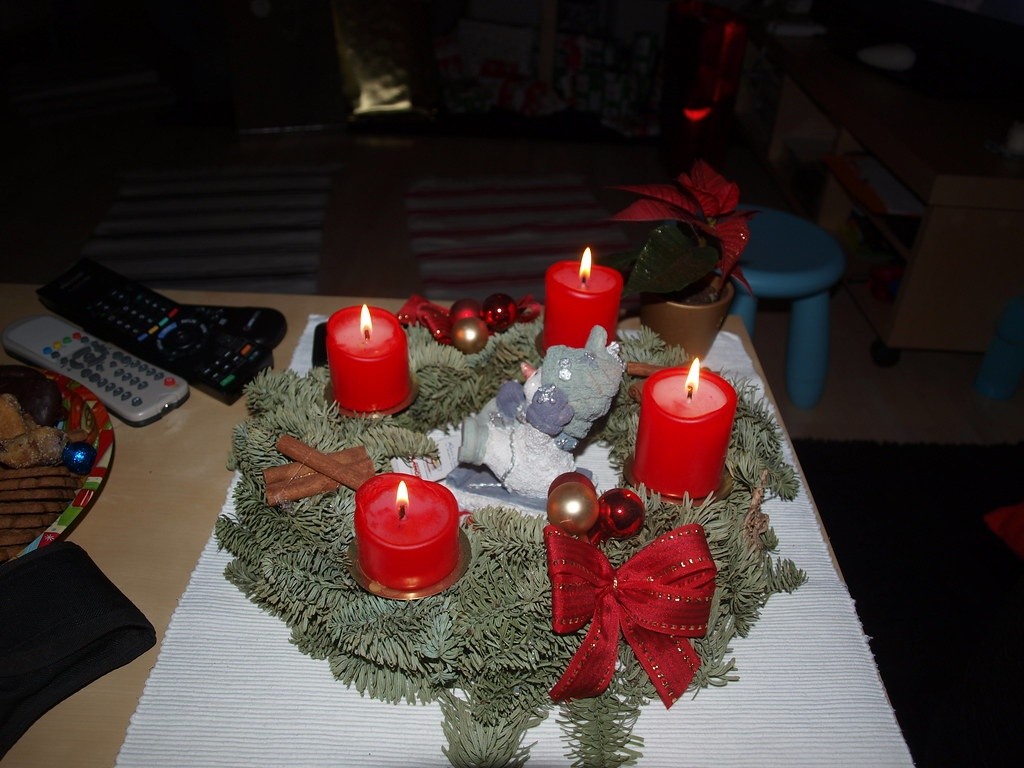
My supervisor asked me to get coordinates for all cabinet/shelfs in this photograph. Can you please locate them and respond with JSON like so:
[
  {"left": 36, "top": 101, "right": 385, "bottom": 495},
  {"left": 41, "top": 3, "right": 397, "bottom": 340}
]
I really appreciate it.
[{"left": 727, "top": 17, "right": 1024, "bottom": 356}]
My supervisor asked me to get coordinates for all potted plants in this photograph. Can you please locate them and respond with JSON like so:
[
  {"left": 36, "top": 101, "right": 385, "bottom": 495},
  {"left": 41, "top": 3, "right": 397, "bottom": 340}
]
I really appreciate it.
[{"left": 588, "top": 157, "right": 761, "bottom": 365}]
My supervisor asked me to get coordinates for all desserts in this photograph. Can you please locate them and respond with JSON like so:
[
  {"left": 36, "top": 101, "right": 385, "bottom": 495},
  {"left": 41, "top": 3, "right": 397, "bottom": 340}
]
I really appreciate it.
[{"left": 0, "top": 363, "right": 72, "bottom": 469}]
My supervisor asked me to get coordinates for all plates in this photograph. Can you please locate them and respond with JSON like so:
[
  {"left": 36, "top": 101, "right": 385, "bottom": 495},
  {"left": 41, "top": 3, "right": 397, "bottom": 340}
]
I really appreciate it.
[{"left": 0, "top": 365, "right": 114, "bottom": 566}]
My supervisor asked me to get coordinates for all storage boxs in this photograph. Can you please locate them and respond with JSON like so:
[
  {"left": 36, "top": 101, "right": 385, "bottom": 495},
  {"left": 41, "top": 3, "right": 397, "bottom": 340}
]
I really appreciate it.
[{"left": 479, "top": 31, "right": 672, "bottom": 139}]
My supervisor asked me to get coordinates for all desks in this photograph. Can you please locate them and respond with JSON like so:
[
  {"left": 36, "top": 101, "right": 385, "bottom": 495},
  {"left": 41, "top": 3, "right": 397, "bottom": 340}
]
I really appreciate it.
[{"left": 0, "top": 280, "right": 918, "bottom": 768}]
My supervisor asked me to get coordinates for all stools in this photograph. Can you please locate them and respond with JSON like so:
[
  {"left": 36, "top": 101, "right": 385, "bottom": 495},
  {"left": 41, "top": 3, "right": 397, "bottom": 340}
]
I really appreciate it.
[{"left": 717, "top": 202, "right": 843, "bottom": 409}]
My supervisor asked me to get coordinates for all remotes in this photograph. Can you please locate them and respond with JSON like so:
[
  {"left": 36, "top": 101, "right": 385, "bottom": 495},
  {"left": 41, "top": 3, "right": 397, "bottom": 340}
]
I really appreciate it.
[{"left": 1, "top": 254, "right": 288, "bottom": 426}]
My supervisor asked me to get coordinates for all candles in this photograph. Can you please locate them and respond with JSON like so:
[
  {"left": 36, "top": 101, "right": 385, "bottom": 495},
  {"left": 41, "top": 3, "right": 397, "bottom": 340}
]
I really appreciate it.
[
  {"left": 353, "top": 474, "right": 460, "bottom": 591},
  {"left": 541, "top": 244, "right": 623, "bottom": 354},
  {"left": 325, "top": 302, "right": 410, "bottom": 413},
  {"left": 631, "top": 354, "right": 738, "bottom": 501}
]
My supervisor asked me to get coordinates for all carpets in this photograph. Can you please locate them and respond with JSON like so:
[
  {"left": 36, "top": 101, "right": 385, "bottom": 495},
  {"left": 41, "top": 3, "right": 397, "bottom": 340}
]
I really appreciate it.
[{"left": 791, "top": 436, "right": 1024, "bottom": 768}]
